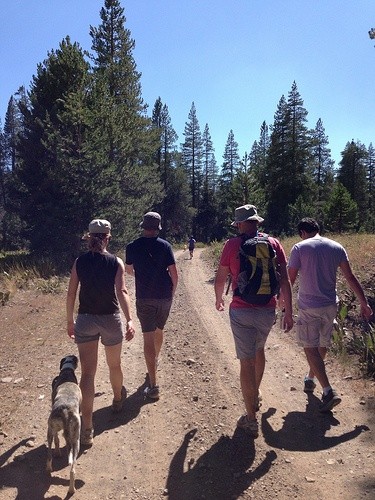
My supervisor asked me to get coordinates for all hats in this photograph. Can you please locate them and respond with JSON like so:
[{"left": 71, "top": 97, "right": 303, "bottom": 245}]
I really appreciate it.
[
  {"left": 231, "top": 204, "right": 265, "bottom": 227},
  {"left": 89, "top": 218, "right": 111, "bottom": 234},
  {"left": 140, "top": 212, "right": 162, "bottom": 231}
]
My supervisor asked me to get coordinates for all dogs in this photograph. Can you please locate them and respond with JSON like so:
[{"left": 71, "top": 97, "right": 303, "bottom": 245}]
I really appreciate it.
[{"left": 46, "top": 355, "right": 82, "bottom": 495}]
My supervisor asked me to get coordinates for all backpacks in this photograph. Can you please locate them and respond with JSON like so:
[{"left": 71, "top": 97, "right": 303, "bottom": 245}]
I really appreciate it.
[{"left": 232, "top": 233, "right": 282, "bottom": 305}]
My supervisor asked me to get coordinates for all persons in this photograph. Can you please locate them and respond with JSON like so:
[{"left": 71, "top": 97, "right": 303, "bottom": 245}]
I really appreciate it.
[
  {"left": 214, "top": 205, "right": 294, "bottom": 436},
  {"left": 124, "top": 212, "right": 179, "bottom": 398},
  {"left": 278, "top": 216, "right": 373, "bottom": 413},
  {"left": 187, "top": 235, "right": 196, "bottom": 260},
  {"left": 65, "top": 219, "right": 135, "bottom": 446}
]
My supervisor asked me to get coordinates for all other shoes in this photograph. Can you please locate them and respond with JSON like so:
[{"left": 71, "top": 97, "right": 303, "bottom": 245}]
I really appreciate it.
[
  {"left": 80, "top": 427, "right": 94, "bottom": 445},
  {"left": 304, "top": 376, "right": 317, "bottom": 392},
  {"left": 145, "top": 373, "right": 150, "bottom": 386},
  {"left": 112, "top": 386, "right": 127, "bottom": 412},
  {"left": 237, "top": 415, "right": 258, "bottom": 433},
  {"left": 319, "top": 390, "right": 342, "bottom": 413},
  {"left": 254, "top": 389, "right": 262, "bottom": 409},
  {"left": 147, "top": 384, "right": 159, "bottom": 401}
]
